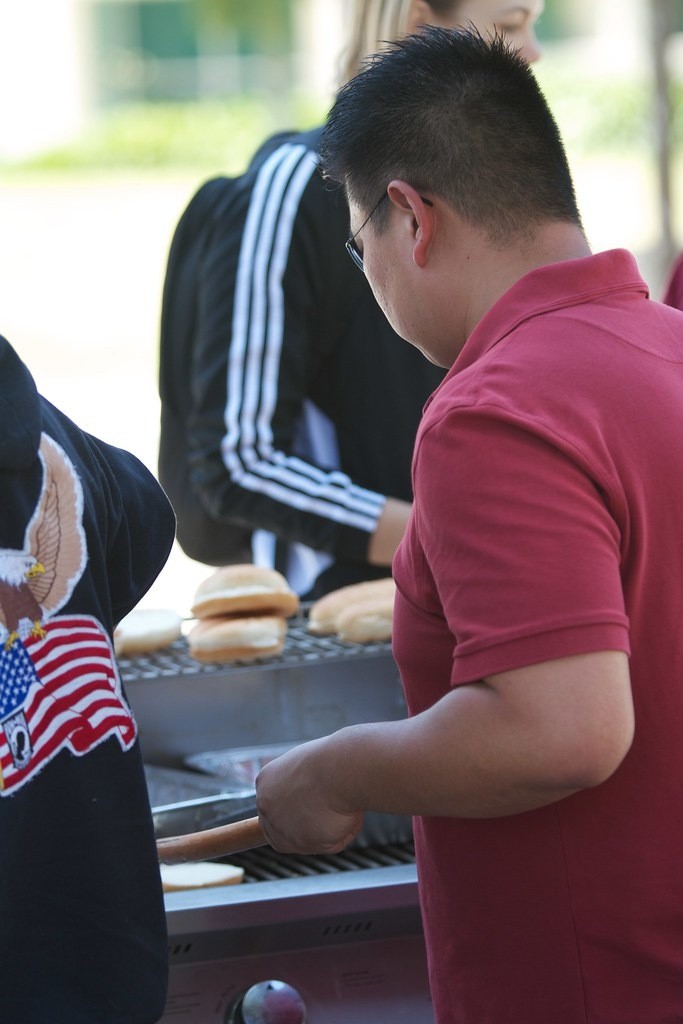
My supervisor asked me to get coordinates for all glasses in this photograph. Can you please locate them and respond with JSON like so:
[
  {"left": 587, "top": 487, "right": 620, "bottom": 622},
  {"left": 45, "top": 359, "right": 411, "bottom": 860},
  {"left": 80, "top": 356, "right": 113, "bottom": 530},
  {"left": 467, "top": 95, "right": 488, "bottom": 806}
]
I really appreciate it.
[{"left": 343, "top": 185, "right": 433, "bottom": 272}]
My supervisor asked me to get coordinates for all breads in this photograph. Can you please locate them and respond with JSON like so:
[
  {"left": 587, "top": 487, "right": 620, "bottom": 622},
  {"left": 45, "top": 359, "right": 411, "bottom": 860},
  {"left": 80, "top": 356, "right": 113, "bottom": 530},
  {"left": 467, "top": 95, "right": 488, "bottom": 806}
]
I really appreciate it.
[
  {"left": 159, "top": 862, "right": 244, "bottom": 894},
  {"left": 113, "top": 563, "right": 396, "bottom": 663}
]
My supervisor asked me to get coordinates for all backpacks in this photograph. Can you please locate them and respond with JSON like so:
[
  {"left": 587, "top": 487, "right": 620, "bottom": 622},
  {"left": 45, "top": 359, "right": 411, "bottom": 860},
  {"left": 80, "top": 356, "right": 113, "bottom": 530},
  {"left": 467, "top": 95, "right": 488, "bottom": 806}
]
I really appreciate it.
[{"left": 158, "top": 132, "right": 347, "bottom": 567}]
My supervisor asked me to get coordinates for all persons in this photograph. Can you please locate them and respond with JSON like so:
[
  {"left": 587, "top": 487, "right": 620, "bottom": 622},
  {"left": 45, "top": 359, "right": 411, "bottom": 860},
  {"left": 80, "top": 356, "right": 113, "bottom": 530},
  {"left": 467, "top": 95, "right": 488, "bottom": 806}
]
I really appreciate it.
[
  {"left": 0, "top": 335, "right": 177, "bottom": 1024},
  {"left": 254, "top": 24, "right": 683, "bottom": 1024},
  {"left": 188, "top": 0, "right": 546, "bottom": 626}
]
